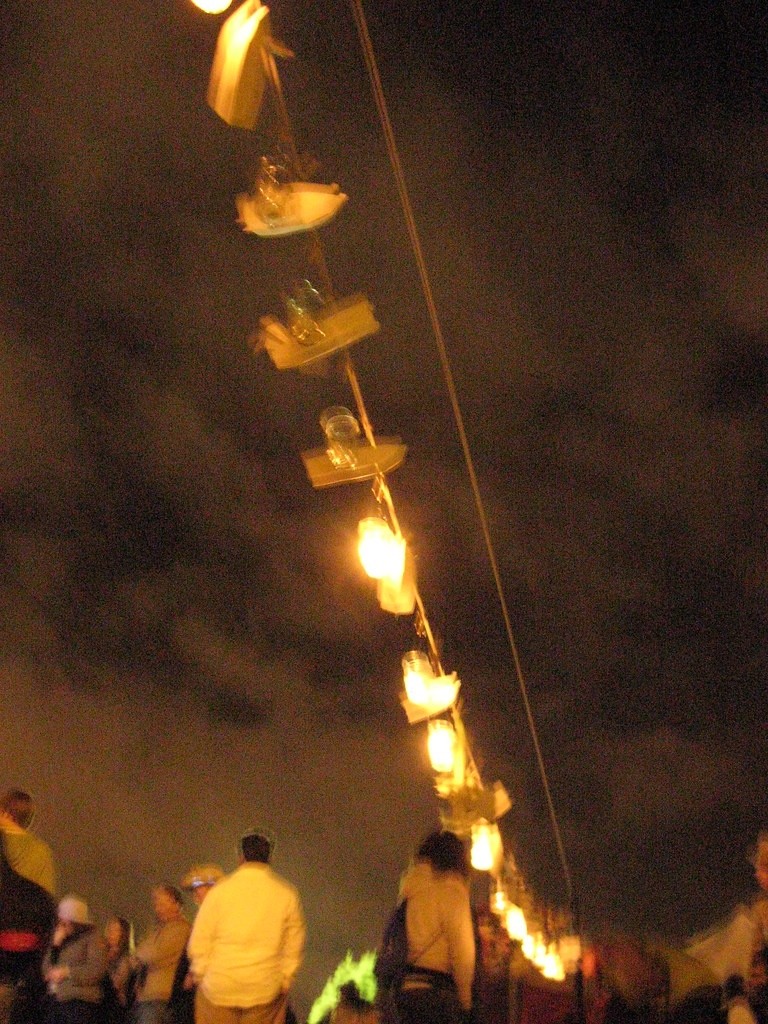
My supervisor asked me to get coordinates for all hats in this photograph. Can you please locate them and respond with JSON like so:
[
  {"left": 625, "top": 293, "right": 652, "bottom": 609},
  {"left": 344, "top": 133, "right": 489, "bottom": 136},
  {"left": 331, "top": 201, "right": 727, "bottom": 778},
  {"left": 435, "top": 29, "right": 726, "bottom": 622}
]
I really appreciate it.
[
  {"left": 58, "top": 896, "right": 97, "bottom": 931},
  {"left": 179, "top": 862, "right": 226, "bottom": 891}
]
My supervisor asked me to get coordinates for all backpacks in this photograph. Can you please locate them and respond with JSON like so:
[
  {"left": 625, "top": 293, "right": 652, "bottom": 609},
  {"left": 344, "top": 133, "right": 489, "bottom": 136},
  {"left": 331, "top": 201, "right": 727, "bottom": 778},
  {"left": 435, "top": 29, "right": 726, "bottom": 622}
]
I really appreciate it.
[{"left": 372, "top": 893, "right": 444, "bottom": 983}]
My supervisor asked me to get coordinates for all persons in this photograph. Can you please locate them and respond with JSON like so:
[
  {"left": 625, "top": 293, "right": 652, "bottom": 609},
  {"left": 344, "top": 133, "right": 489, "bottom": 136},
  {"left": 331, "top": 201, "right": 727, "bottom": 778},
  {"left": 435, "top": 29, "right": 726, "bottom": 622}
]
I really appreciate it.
[
  {"left": 1, "top": 789, "right": 768, "bottom": 1024},
  {"left": 188, "top": 835, "right": 306, "bottom": 1023}
]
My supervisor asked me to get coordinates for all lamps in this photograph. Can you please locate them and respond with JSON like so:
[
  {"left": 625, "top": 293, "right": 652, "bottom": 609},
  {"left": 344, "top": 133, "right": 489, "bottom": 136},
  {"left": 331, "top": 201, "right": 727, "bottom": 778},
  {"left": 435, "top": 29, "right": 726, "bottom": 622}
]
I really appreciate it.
[
  {"left": 470, "top": 819, "right": 568, "bottom": 982},
  {"left": 353, "top": 510, "right": 409, "bottom": 590},
  {"left": 234, "top": 155, "right": 349, "bottom": 238},
  {"left": 258, "top": 282, "right": 377, "bottom": 372},
  {"left": 298, "top": 406, "right": 405, "bottom": 489},
  {"left": 402, "top": 645, "right": 459, "bottom": 728},
  {"left": 426, "top": 718, "right": 456, "bottom": 777}
]
